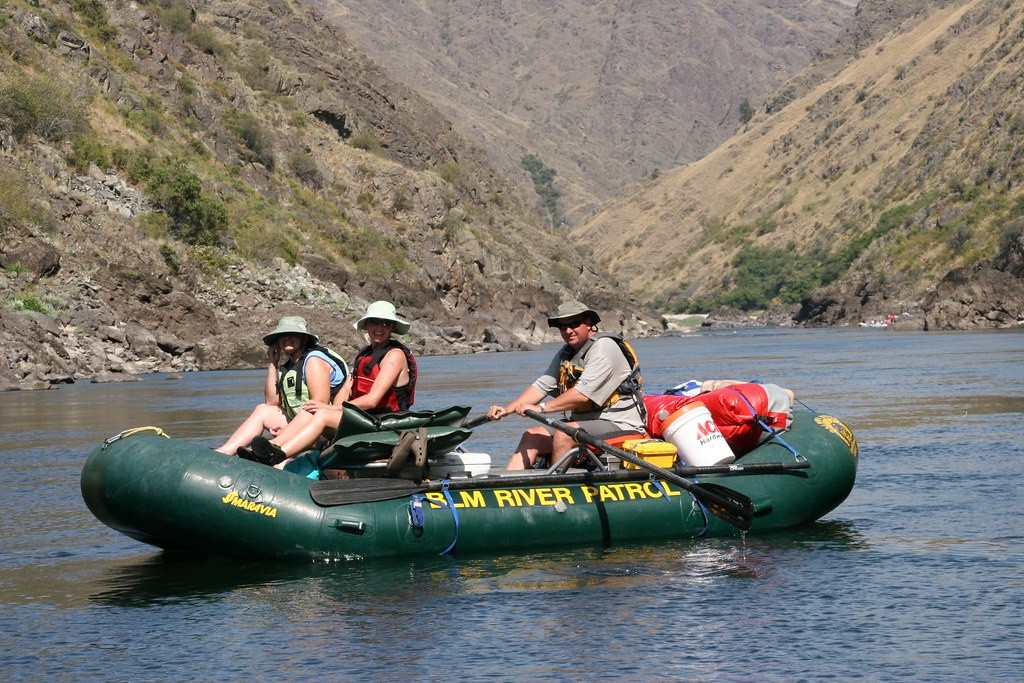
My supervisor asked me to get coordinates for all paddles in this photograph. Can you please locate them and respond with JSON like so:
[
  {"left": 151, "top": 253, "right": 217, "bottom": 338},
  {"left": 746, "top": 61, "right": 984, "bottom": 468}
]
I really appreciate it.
[
  {"left": 520, "top": 408, "right": 753, "bottom": 530},
  {"left": 310, "top": 460, "right": 812, "bottom": 507}
]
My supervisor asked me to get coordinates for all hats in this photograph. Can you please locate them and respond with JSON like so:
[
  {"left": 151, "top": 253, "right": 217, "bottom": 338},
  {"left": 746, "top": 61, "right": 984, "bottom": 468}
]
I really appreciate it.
[
  {"left": 549, "top": 301, "right": 601, "bottom": 328},
  {"left": 353, "top": 300, "right": 410, "bottom": 331},
  {"left": 263, "top": 316, "right": 318, "bottom": 347}
]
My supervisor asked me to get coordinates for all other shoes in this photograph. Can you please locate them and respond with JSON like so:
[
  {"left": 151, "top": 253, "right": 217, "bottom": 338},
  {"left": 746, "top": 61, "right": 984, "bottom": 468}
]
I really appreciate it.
[{"left": 236, "top": 437, "right": 285, "bottom": 465}]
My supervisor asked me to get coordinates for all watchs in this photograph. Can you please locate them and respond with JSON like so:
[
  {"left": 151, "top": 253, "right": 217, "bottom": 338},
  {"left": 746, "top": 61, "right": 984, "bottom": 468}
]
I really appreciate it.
[{"left": 539, "top": 402, "right": 545, "bottom": 413}]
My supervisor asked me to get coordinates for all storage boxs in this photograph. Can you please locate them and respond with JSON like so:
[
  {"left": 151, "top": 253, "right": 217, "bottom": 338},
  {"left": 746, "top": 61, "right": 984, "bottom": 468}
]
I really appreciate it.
[
  {"left": 428, "top": 453, "right": 491, "bottom": 478},
  {"left": 621, "top": 438, "right": 676, "bottom": 469}
]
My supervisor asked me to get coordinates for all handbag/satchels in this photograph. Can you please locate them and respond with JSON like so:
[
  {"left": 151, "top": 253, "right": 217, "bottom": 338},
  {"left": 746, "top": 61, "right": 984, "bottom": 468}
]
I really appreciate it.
[{"left": 318, "top": 469, "right": 350, "bottom": 481}]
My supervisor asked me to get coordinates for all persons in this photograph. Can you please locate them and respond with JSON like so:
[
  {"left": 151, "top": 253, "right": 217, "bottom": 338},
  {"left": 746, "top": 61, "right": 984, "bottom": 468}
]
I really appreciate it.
[
  {"left": 486, "top": 300, "right": 646, "bottom": 473},
  {"left": 237, "top": 300, "right": 418, "bottom": 465},
  {"left": 214, "top": 316, "right": 350, "bottom": 456}
]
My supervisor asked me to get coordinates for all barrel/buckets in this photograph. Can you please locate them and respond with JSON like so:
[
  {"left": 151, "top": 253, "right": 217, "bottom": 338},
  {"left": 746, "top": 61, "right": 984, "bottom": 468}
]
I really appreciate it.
[{"left": 659, "top": 401, "right": 735, "bottom": 466}]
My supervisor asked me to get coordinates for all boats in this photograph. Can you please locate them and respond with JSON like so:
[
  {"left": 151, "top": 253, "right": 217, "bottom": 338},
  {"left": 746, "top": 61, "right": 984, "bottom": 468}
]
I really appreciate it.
[{"left": 80, "top": 410, "right": 862, "bottom": 553}]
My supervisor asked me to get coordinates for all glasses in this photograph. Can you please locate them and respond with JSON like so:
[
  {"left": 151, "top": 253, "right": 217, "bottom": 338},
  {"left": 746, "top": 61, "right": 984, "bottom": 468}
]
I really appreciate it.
[
  {"left": 367, "top": 319, "right": 393, "bottom": 329},
  {"left": 557, "top": 319, "right": 585, "bottom": 330}
]
